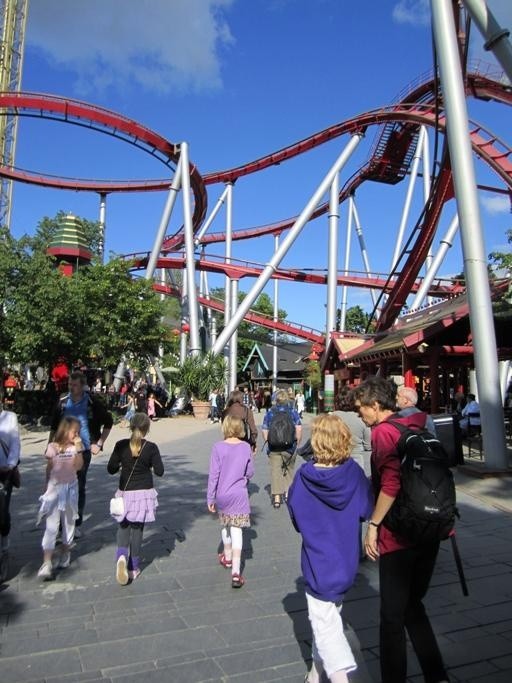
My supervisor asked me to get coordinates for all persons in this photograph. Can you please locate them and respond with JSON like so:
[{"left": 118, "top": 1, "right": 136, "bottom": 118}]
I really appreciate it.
[
  {"left": 396, "top": 387, "right": 437, "bottom": 437},
  {"left": 93, "top": 377, "right": 102, "bottom": 393},
  {"left": 261, "top": 389, "right": 303, "bottom": 509},
  {"left": 50, "top": 371, "right": 112, "bottom": 545},
  {"left": 109, "top": 373, "right": 164, "bottom": 427},
  {"left": 0, "top": 400, "right": 22, "bottom": 582},
  {"left": 346, "top": 374, "right": 450, "bottom": 683},
  {"left": 206, "top": 414, "right": 254, "bottom": 587},
  {"left": 106, "top": 412, "right": 165, "bottom": 585},
  {"left": 2, "top": 369, "right": 46, "bottom": 391},
  {"left": 453, "top": 377, "right": 481, "bottom": 440},
  {"left": 285, "top": 412, "right": 377, "bottom": 683},
  {"left": 208, "top": 385, "right": 306, "bottom": 454},
  {"left": 36, "top": 416, "right": 86, "bottom": 578}
]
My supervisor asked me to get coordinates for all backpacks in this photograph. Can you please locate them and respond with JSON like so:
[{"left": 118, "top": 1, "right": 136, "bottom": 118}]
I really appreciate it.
[
  {"left": 370, "top": 419, "right": 456, "bottom": 545},
  {"left": 267, "top": 407, "right": 296, "bottom": 450}
]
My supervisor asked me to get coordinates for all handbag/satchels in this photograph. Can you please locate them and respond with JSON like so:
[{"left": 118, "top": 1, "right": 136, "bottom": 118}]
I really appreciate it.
[
  {"left": 8, "top": 464, "right": 21, "bottom": 489},
  {"left": 240, "top": 423, "right": 256, "bottom": 446},
  {"left": 109, "top": 498, "right": 125, "bottom": 517}
]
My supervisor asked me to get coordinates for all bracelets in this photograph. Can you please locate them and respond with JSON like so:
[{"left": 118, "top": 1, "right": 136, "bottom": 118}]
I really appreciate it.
[
  {"left": 369, "top": 521, "right": 380, "bottom": 527},
  {"left": 76, "top": 450, "right": 82, "bottom": 453},
  {"left": 96, "top": 443, "right": 102, "bottom": 448}
]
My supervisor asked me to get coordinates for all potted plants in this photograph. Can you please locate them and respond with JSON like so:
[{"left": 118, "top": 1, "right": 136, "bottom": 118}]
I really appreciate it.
[{"left": 175, "top": 350, "right": 232, "bottom": 420}]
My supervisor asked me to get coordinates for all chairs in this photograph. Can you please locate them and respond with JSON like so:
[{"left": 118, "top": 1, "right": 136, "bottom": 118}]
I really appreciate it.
[{"left": 460, "top": 412, "right": 483, "bottom": 460}]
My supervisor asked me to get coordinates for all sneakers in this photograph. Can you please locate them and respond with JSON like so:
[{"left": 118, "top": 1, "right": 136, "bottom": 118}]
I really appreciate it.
[
  {"left": 1, "top": 535, "right": 9, "bottom": 551},
  {"left": 116, "top": 555, "right": 129, "bottom": 586},
  {"left": 55, "top": 532, "right": 62, "bottom": 544},
  {"left": 128, "top": 568, "right": 140, "bottom": 580},
  {"left": 55, "top": 549, "right": 71, "bottom": 569},
  {"left": 74, "top": 527, "right": 82, "bottom": 539},
  {"left": 37, "top": 560, "right": 53, "bottom": 578}
]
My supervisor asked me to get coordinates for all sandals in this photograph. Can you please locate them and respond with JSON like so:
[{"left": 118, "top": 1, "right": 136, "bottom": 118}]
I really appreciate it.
[
  {"left": 274, "top": 499, "right": 283, "bottom": 509},
  {"left": 218, "top": 553, "right": 232, "bottom": 569},
  {"left": 232, "top": 573, "right": 245, "bottom": 588}
]
